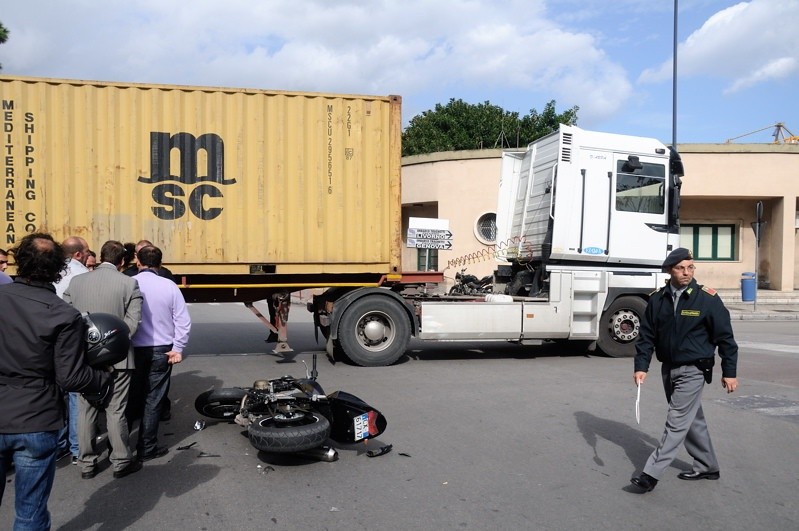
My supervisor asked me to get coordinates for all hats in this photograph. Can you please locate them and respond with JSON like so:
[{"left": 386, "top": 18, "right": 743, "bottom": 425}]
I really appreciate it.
[{"left": 659, "top": 248, "right": 695, "bottom": 276}]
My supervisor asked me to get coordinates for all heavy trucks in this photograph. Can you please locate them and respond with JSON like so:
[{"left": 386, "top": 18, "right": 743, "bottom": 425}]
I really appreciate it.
[{"left": 1, "top": 71, "right": 686, "bottom": 368}]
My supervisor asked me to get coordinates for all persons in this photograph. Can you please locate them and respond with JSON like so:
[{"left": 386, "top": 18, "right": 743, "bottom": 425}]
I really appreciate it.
[
  {"left": 53, "top": 237, "right": 96, "bottom": 464},
  {"left": 0, "top": 248, "right": 9, "bottom": 272},
  {"left": 630, "top": 248, "right": 739, "bottom": 490},
  {"left": 0, "top": 233, "right": 114, "bottom": 531},
  {"left": 131, "top": 246, "right": 192, "bottom": 458},
  {"left": 63, "top": 240, "right": 145, "bottom": 478},
  {"left": 119, "top": 241, "right": 174, "bottom": 420}
]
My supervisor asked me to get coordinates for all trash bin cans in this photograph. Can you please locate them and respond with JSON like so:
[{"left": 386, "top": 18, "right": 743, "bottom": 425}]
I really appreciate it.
[{"left": 741, "top": 272, "right": 755, "bottom": 301}]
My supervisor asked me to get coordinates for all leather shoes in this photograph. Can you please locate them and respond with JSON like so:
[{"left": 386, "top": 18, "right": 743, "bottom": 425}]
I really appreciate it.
[
  {"left": 677, "top": 467, "right": 724, "bottom": 481},
  {"left": 631, "top": 472, "right": 659, "bottom": 492}
]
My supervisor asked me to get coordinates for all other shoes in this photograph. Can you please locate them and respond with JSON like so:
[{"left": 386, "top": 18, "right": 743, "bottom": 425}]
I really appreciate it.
[
  {"left": 161, "top": 413, "right": 171, "bottom": 421},
  {"left": 55, "top": 449, "right": 71, "bottom": 462},
  {"left": 71, "top": 456, "right": 80, "bottom": 465},
  {"left": 113, "top": 460, "right": 143, "bottom": 478},
  {"left": 81, "top": 462, "right": 99, "bottom": 479},
  {"left": 138, "top": 446, "right": 170, "bottom": 464}
]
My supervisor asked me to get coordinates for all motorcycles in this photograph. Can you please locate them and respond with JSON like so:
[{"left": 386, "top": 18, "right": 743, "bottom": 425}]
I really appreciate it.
[{"left": 194, "top": 352, "right": 388, "bottom": 463}]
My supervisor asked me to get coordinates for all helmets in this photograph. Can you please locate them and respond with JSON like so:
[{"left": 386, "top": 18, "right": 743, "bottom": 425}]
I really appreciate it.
[{"left": 81, "top": 311, "right": 134, "bottom": 373}]
